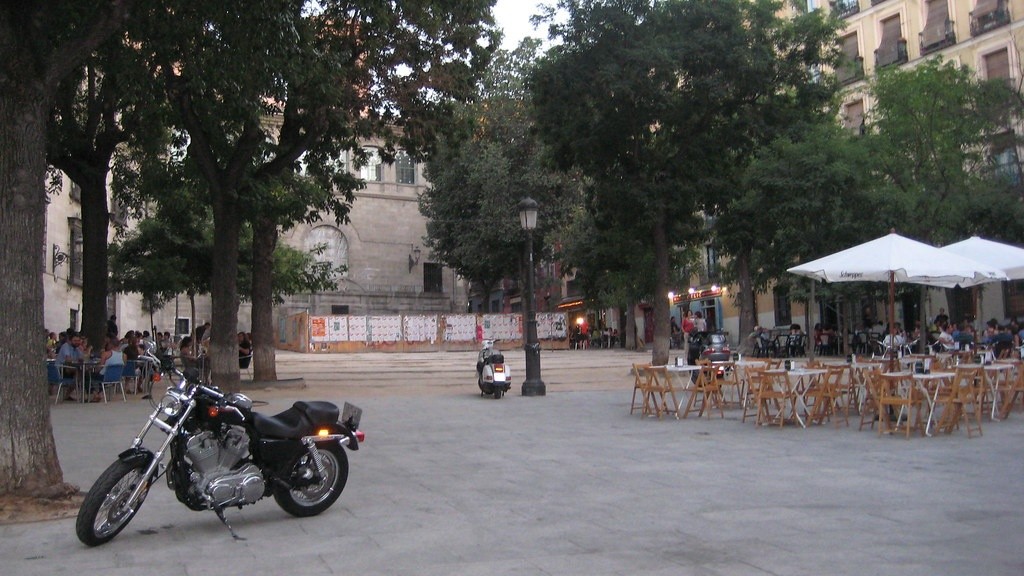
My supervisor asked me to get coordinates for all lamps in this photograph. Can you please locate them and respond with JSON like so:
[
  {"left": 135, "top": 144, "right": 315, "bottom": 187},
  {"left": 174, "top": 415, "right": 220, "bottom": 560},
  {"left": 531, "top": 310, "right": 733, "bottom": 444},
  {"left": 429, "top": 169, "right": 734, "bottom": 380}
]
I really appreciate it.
[{"left": 409, "top": 246, "right": 421, "bottom": 274}]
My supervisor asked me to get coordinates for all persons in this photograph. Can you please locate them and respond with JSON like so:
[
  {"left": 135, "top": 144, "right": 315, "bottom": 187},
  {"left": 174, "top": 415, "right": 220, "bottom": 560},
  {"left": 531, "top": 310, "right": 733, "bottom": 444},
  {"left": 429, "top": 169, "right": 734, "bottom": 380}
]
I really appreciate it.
[
  {"left": 670, "top": 316, "right": 679, "bottom": 334},
  {"left": 569, "top": 325, "right": 619, "bottom": 348},
  {"left": 882, "top": 308, "right": 1024, "bottom": 360},
  {"left": 180, "top": 322, "right": 211, "bottom": 385},
  {"left": 812, "top": 319, "right": 885, "bottom": 354},
  {"left": 746, "top": 324, "right": 805, "bottom": 356},
  {"left": 106, "top": 313, "right": 178, "bottom": 397},
  {"left": 238, "top": 331, "right": 253, "bottom": 357},
  {"left": 681, "top": 310, "right": 708, "bottom": 357},
  {"left": 43, "top": 327, "right": 127, "bottom": 402}
]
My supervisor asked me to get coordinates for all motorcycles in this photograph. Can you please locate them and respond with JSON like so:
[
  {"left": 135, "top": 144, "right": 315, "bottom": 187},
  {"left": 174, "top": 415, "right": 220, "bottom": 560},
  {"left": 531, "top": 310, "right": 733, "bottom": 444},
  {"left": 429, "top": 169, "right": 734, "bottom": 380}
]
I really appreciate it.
[{"left": 75, "top": 338, "right": 365, "bottom": 548}]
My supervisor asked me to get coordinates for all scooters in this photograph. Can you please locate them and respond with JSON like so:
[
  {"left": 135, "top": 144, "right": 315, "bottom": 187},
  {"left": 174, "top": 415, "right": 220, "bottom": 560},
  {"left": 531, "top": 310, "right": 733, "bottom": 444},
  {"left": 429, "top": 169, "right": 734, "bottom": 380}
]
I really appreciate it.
[
  {"left": 685, "top": 324, "right": 740, "bottom": 392},
  {"left": 476, "top": 336, "right": 512, "bottom": 399}
]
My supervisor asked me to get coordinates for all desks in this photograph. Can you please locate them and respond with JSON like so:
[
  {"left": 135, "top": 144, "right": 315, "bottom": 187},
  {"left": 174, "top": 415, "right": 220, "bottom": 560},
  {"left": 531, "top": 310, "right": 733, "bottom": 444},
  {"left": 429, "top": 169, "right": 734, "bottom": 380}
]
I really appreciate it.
[
  {"left": 883, "top": 358, "right": 922, "bottom": 395},
  {"left": 649, "top": 365, "right": 715, "bottom": 421},
  {"left": 881, "top": 370, "right": 957, "bottom": 437},
  {"left": 952, "top": 364, "right": 1013, "bottom": 421},
  {"left": 712, "top": 361, "right": 765, "bottom": 409},
  {"left": 47, "top": 357, "right": 101, "bottom": 403},
  {"left": 823, "top": 360, "right": 884, "bottom": 415},
  {"left": 762, "top": 368, "right": 828, "bottom": 429}
]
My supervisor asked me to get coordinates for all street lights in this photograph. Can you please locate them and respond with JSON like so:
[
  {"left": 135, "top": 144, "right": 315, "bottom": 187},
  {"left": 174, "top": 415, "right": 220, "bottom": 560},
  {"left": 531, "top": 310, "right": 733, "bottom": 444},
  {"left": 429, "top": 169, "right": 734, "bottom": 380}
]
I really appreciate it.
[{"left": 515, "top": 195, "right": 546, "bottom": 398}]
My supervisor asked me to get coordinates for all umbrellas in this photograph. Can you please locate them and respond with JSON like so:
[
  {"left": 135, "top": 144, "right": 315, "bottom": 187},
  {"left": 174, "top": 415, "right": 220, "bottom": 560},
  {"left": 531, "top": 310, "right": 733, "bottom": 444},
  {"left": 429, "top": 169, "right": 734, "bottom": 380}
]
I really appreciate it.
[
  {"left": 784, "top": 230, "right": 1010, "bottom": 415},
  {"left": 936, "top": 234, "right": 1024, "bottom": 385}
]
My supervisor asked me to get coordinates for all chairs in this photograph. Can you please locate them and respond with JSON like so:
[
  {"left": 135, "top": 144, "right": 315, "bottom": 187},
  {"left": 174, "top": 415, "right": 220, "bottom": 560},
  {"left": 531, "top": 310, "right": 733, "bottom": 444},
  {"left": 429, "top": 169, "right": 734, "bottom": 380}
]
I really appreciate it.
[
  {"left": 630, "top": 333, "right": 1024, "bottom": 440},
  {"left": 46, "top": 347, "right": 211, "bottom": 405},
  {"left": 239, "top": 354, "right": 253, "bottom": 380}
]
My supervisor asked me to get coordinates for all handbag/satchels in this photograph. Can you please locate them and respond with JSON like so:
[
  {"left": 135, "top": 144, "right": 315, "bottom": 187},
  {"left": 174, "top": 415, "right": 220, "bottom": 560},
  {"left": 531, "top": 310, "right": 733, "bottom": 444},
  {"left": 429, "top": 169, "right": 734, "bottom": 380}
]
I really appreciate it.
[{"left": 932, "top": 340, "right": 949, "bottom": 353}]
